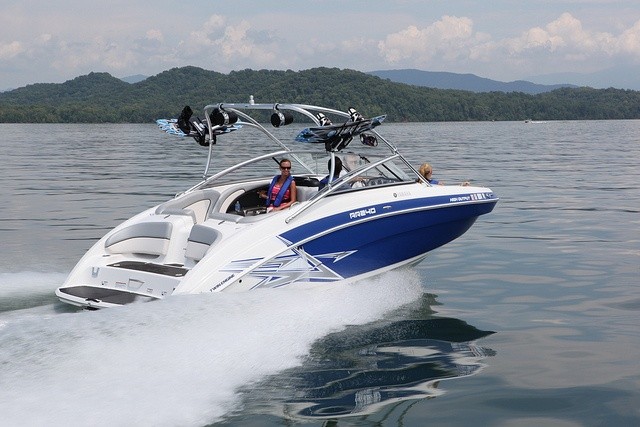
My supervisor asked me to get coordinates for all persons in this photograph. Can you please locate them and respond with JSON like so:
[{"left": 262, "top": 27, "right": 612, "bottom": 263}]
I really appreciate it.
[
  {"left": 416, "top": 163, "right": 471, "bottom": 187},
  {"left": 318, "top": 154, "right": 363, "bottom": 194},
  {"left": 266, "top": 158, "right": 297, "bottom": 214}
]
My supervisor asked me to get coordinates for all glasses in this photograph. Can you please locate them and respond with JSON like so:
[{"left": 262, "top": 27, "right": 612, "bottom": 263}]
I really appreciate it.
[{"left": 281, "top": 166, "right": 291, "bottom": 170}]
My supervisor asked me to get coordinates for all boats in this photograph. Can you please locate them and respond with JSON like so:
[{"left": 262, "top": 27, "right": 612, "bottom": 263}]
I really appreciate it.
[
  {"left": 246, "top": 336, "right": 496, "bottom": 427},
  {"left": 53, "top": 92, "right": 501, "bottom": 316}
]
